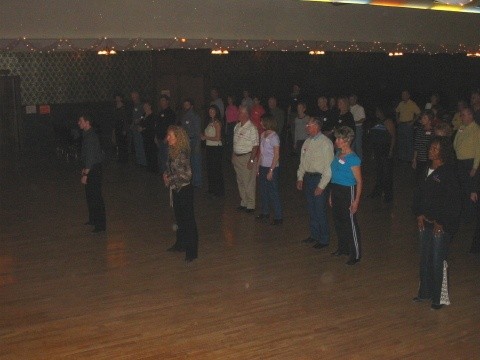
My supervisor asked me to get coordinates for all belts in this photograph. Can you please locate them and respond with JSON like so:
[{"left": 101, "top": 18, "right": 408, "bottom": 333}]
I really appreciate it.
[{"left": 235, "top": 151, "right": 252, "bottom": 156}]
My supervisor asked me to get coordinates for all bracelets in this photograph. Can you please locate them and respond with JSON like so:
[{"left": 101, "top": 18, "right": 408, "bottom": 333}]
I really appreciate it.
[
  {"left": 270, "top": 168, "right": 273, "bottom": 171},
  {"left": 82, "top": 172, "right": 89, "bottom": 176}
]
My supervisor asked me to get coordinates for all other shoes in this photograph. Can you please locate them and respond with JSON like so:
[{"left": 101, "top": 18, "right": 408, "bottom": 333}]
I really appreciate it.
[
  {"left": 255, "top": 214, "right": 270, "bottom": 222},
  {"left": 302, "top": 236, "right": 314, "bottom": 243},
  {"left": 312, "top": 242, "right": 329, "bottom": 249},
  {"left": 431, "top": 304, "right": 444, "bottom": 310},
  {"left": 271, "top": 218, "right": 284, "bottom": 225},
  {"left": 332, "top": 251, "right": 350, "bottom": 257},
  {"left": 167, "top": 244, "right": 185, "bottom": 253},
  {"left": 345, "top": 259, "right": 360, "bottom": 266},
  {"left": 86, "top": 221, "right": 106, "bottom": 233},
  {"left": 235, "top": 205, "right": 256, "bottom": 215}
]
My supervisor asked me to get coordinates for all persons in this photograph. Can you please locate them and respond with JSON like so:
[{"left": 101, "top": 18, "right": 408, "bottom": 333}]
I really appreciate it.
[
  {"left": 412, "top": 136, "right": 461, "bottom": 310},
  {"left": 109, "top": 89, "right": 480, "bottom": 264},
  {"left": 77, "top": 112, "right": 107, "bottom": 233},
  {"left": 162, "top": 124, "right": 197, "bottom": 261}
]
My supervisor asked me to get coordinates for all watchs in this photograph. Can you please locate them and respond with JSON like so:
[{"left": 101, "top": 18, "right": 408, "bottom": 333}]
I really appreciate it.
[{"left": 249, "top": 160, "right": 254, "bottom": 163}]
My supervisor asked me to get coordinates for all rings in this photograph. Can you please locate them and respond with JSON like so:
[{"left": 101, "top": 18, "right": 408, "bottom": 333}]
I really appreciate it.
[{"left": 438, "top": 230, "right": 440, "bottom": 232}]
